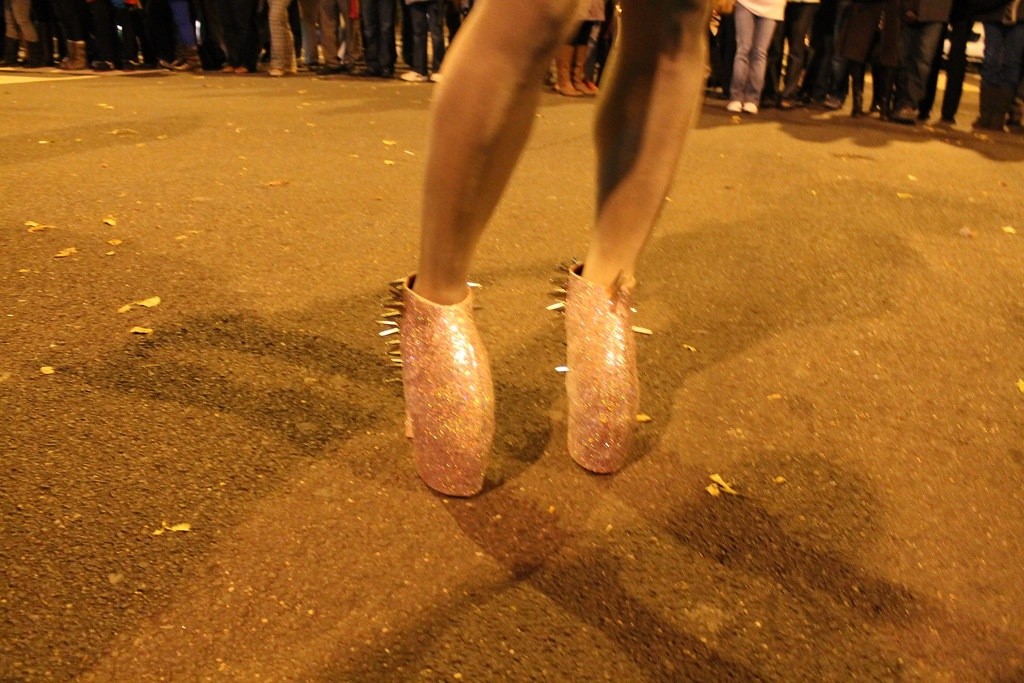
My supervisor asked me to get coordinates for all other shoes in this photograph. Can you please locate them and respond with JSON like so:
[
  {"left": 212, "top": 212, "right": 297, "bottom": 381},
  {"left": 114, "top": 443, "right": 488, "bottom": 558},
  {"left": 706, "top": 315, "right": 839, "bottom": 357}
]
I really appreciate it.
[
  {"left": 0, "top": 34, "right": 441, "bottom": 83},
  {"left": 711, "top": 84, "right": 1006, "bottom": 131},
  {"left": 547, "top": 68, "right": 600, "bottom": 96},
  {"left": 546, "top": 257, "right": 640, "bottom": 473},
  {"left": 373, "top": 271, "right": 496, "bottom": 498}
]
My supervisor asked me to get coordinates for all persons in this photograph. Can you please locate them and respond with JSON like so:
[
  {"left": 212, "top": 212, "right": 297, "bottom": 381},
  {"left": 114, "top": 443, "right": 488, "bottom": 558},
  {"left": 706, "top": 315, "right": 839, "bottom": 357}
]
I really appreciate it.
[
  {"left": 0, "top": 0, "right": 1024, "bottom": 133},
  {"left": 375, "top": 0, "right": 712, "bottom": 497}
]
